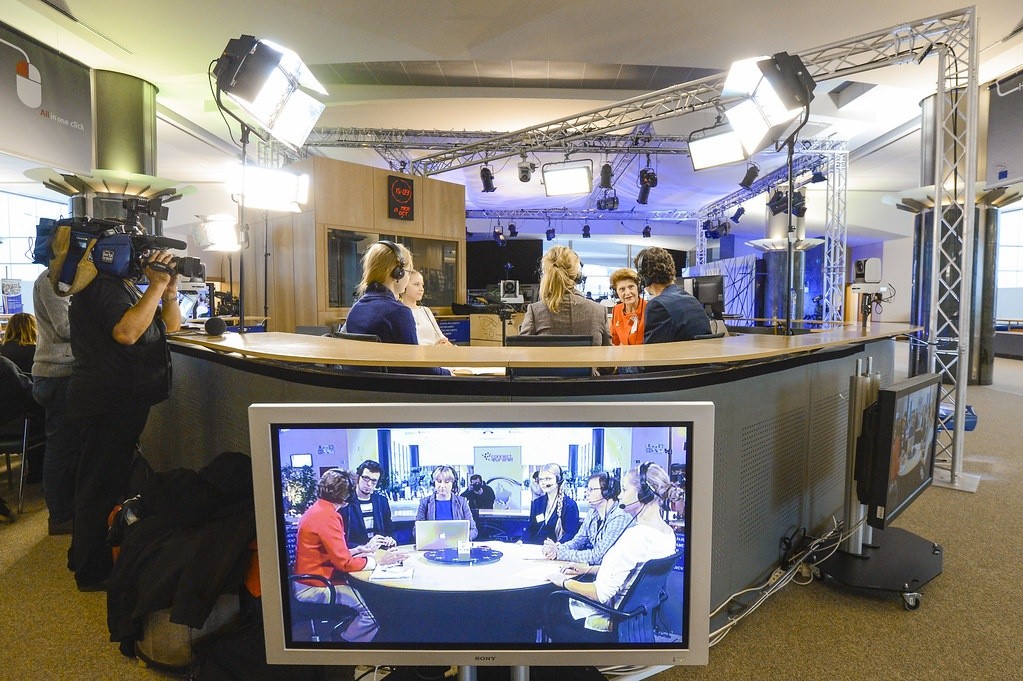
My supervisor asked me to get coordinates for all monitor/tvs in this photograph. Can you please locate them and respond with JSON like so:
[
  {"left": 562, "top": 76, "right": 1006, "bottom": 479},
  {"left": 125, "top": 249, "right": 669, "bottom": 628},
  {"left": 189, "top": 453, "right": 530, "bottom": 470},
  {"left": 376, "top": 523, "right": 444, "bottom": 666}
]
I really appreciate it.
[
  {"left": 855, "top": 373, "right": 941, "bottom": 532},
  {"left": 247, "top": 401, "right": 715, "bottom": 666},
  {"left": 136, "top": 282, "right": 216, "bottom": 320},
  {"left": 674, "top": 275, "right": 726, "bottom": 322}
]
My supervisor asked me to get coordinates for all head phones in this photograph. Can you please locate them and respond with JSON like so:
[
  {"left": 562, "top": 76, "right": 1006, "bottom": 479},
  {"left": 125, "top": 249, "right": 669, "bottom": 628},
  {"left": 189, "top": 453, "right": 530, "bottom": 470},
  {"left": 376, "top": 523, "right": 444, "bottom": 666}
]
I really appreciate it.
[
  {"left": 612, "top": 281, "right": 642, "bottom": 299},
  {"left": 536, "top": 465, "right": 564, "bottom": 486},
  {"left": 357, "top": 460, "right": 384, "bottom": 489},
  {"left": 637, "top": 253, "right": 652, "bottom": 286},
  {"left": 573, "top": 252, "right": 587, "bottom": 284},
  {"left": 430, "top": 466, "right": 458, "bottom": 489},
  {"left": 329, "top": 469, "right": 356, "bottom": 504},
  {"left": 601, "top": 472, "right": 615, "bottom": 500},
  {"left": 637, "top": 461, "right": 655, "bottom": 504},
  {"left": 378, "top": 240, "right": 405, "bottom": 283}
]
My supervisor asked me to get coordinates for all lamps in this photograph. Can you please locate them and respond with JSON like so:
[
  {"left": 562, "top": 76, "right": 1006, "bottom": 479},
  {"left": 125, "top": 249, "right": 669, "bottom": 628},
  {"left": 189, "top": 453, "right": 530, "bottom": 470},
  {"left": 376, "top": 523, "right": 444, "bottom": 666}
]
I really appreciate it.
[
  {"left": 479, "top": 162, "right": 498, "bottom": 193},
  {"left": 493, "top": 218, "right": 506, "bottom": 246},
  {"left": 703, "top": 50, "right": 828, "bottom": 336},
  {"left": 686, "top": 114, "right": 749, "bottom": 172},
  {"left": 598, "top": 154, "right": 613, "bottom": 191},
  {"left": 508, "top": 219, "right": 518, "bottom": 237},
  {"left": 545, "top": 220, "right": 556, "bottom": 241},
  {"left": 540, "top": 153, "right": 593, "bottom": 198},
  {"left": 517, "top": 156, "right": 535, "bottom": 183},
  {"left": 642, "top": 225, "right": 652, "bottom": 238},
  {"left": 582, "top": 221, "right": 591, "bottom": 238},
  {"left": 596, "top": 183, "right": 619, "bottom": 211},
  {"left": 194, "top": 33, "right": 329, "bottom": 334},
  {"left": 635, "top": 152, "right": 659, "bottom": 204}
]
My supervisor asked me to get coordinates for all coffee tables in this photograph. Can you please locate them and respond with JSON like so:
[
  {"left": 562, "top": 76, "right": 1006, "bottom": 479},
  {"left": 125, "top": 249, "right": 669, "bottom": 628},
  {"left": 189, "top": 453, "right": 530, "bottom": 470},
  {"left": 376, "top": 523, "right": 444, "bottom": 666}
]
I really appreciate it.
[{"left": 995, "top": 318, "right": 1023, "bottom": 331}]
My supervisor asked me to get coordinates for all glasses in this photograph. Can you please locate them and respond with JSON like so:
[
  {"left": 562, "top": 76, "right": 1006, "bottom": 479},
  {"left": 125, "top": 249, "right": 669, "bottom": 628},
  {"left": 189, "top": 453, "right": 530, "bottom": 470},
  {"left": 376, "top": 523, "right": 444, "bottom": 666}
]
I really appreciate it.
[
  {"left": 586, "top": 487, "right": 602, "bottom": 493},
  {"left": 361, "top": 475, "right": 378, "bottom": 484}
]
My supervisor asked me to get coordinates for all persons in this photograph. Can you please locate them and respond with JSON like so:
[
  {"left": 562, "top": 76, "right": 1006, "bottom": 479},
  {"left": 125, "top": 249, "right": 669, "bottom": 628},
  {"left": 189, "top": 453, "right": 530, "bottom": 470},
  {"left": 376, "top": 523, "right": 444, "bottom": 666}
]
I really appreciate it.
[
  {"left": 0, "top": 313, "right": 47, "bottom": 486},
  {"left": 518, "top": 246, "right": 616, "bottom": 376},
  {"left": 64, "top": 218, "right": 182, "bottom": 573},
  {"left": 544, "top": 461, "right": 677, "bottom": 636},
  {"left": 337, "top": 460, "right": 398, "bottom": 555},
  {"left": 398, "top": 268, "right": 458, "bottom": 347},
  {"left": 634, "top": 246, "right": 713, "bottom": 373},
  {"left": 31, "top": 268, "right": 84, "bottom": 536},
  {"left": 346, "top": 240, "right": 456, "bottom": 378},
  {"left": 658, "top": 482, "right": 685, "bottom": 533},
  {"left": 516, "top": 462, "right": 579, "bottom": 545},
  {"left": 887, "top": 400, "right": 936, "bottom": 511},
  {"left": 458, "top": 474, "right": 496, "bottom": 509},
  {"left": 291, "top": 469, "right": 379, "bottom": 642},
  {"left": 411, "top": 465, "right": 479, "bottom": 543},
  {"left": 608, "top": 267, "right": 648, "bottom": 376},
  {"left": 542, "top": 471, "right": 637, "bottom": 565}
]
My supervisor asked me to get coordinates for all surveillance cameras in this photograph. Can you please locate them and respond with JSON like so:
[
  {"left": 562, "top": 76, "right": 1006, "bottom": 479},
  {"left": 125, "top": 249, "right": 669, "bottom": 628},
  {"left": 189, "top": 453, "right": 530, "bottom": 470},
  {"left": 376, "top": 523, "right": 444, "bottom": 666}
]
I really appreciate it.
[
  {"left": 853, "top": 256, "right": 882, "bottom": 284},
  {"left": 500, "top": 280, "right": 519, "bottom": 299},
  {"left": 518, "top": 161, "right": 531, "bottom": 182}
]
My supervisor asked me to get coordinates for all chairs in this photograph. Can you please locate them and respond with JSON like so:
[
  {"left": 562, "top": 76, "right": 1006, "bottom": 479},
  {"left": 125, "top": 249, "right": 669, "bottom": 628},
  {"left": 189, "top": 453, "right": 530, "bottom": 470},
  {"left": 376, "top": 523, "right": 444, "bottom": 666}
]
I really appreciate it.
[
  {"left": 539, "top": 546, "right": 685, "bottom": 643},
  {"left": 0, "top": 355, "right": 51, "bottom": 516},
  {"left": 287, "top": 574, "right": 359, "bottom": 644}
]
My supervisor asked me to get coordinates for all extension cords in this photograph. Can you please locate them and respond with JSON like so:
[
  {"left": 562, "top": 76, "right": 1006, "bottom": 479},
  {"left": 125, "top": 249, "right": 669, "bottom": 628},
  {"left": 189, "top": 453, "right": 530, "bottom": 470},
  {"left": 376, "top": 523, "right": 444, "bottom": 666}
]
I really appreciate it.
[{"left": 768, "top": 565, "right": 793, "bottom": 586}]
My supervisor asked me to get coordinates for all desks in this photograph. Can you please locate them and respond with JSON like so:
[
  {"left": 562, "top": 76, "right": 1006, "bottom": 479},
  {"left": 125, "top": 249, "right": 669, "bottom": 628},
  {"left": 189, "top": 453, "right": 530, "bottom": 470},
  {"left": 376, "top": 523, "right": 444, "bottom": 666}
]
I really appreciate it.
[{"left": 347, "top": 541, "right": 590, "bottom": 643}]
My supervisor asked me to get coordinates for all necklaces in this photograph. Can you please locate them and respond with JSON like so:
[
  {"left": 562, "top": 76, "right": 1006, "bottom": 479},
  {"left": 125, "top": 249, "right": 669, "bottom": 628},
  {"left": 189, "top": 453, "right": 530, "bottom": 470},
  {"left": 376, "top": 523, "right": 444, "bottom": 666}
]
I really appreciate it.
[{"left": 624, "top": 312, "right": 627, "bottom": 314}]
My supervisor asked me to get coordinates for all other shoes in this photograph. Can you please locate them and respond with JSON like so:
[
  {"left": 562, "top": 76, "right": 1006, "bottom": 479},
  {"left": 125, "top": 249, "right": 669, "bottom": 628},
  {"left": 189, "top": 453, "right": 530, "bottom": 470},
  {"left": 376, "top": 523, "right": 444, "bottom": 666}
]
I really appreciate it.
[
  {"left": 75, "top": 573, "right": 108, "bottom": 592},
  {"left": 48, "top": 518, "right": 74, "bottom": 534}
]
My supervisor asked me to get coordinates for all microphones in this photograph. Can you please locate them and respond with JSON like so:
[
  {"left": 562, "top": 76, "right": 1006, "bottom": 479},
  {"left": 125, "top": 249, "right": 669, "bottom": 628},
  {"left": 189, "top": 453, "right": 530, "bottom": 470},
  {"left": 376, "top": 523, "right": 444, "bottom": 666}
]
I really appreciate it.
[
  {"left": 619, "top": 495, "right": 648, "bottom": 510},
  {"left": 639, "top": 282, "right": 643, "bottom": 293},
  {"left": 205, "top": 318, "right": 227, "bottom": 335},
  {"left": 155, "top": 236, "right": 187, "bottom": 250},
  {"left": 546, "top": 481, "right": 562, "bottom": 487},
  {"left": 589, "top": 494, "right": 610, "bottom": 503}
]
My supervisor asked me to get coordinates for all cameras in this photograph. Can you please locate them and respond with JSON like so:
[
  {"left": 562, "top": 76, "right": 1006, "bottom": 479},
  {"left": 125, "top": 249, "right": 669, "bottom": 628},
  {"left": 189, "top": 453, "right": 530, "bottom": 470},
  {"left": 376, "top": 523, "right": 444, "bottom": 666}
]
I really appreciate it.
[{"left": 470, "top": 482, "right": 482, "bottom": 492}]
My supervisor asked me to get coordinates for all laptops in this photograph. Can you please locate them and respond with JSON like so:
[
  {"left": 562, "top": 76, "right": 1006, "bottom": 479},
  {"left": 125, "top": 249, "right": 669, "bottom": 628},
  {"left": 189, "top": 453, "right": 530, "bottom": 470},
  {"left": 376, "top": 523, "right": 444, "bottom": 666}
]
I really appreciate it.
[{"left": 415, "top": 520, "right": 470, "bottom": 551}]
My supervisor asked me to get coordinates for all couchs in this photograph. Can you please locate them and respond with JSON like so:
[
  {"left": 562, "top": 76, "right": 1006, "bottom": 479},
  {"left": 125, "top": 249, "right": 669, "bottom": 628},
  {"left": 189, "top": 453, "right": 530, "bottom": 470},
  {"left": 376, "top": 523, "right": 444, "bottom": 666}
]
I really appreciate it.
[{"left": 995, "top": 324, "right": 1023, "bottom": 360}]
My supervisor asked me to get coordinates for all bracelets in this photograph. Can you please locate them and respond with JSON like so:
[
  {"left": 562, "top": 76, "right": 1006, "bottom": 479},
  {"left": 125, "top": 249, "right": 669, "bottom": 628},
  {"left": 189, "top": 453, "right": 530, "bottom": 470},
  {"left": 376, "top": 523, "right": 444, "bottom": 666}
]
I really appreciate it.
[
  {"left": 161, "top": 295, "right": 177, "bottom": 303},
  {"left": 563, "top": 578, "right": 570, "bottom": 588}
]
